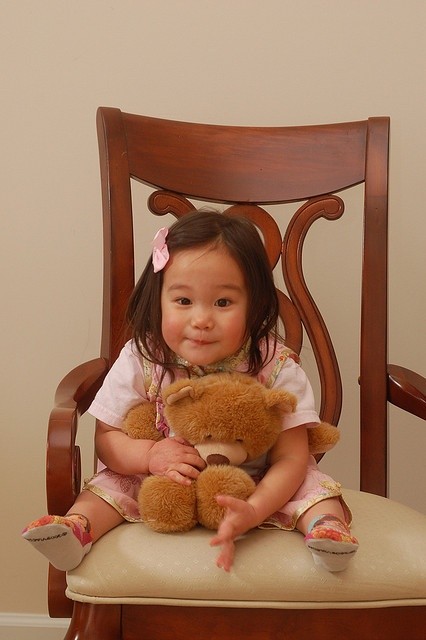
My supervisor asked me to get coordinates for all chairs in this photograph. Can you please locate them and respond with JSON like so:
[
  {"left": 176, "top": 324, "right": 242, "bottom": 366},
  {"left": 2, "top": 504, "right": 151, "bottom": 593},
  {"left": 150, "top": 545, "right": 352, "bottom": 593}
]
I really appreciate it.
[{"left": 44, "top": 107, "right": 426, "bottom": 640}]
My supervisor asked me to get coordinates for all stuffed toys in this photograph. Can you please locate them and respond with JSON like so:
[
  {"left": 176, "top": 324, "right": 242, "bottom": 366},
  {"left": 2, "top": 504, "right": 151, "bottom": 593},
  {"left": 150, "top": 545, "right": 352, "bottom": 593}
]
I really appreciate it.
[{"left": 122, "top": 371, "right": 341, "bottom": 534}]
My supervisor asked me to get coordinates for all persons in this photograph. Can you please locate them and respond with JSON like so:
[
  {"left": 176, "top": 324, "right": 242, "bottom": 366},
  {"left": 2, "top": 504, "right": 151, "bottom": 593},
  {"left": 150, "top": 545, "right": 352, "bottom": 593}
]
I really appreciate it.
[{"left": 18, "top": 205, "right": 360, "bottom": 575}]
